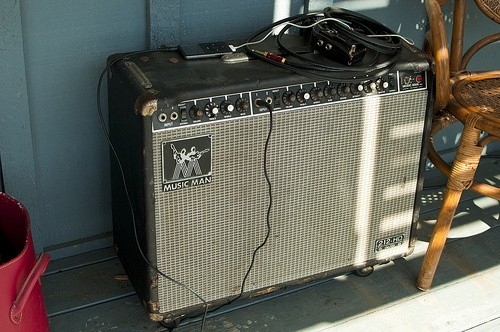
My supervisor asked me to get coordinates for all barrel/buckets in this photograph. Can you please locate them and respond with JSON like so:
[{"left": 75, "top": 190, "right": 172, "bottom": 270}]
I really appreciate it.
[{"left": 1, "top": 191, "right": 48, "bottom": 331}]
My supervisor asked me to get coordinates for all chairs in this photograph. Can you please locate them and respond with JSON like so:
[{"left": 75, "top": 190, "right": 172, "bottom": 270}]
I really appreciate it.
[{"left": 415, "top": 0, "right": 500, "bottom": 293}]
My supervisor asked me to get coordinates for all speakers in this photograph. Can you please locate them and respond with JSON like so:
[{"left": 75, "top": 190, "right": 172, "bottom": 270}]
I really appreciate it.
[{"left": 105, "top": 28, "right": 435, "bottom": 323}]
